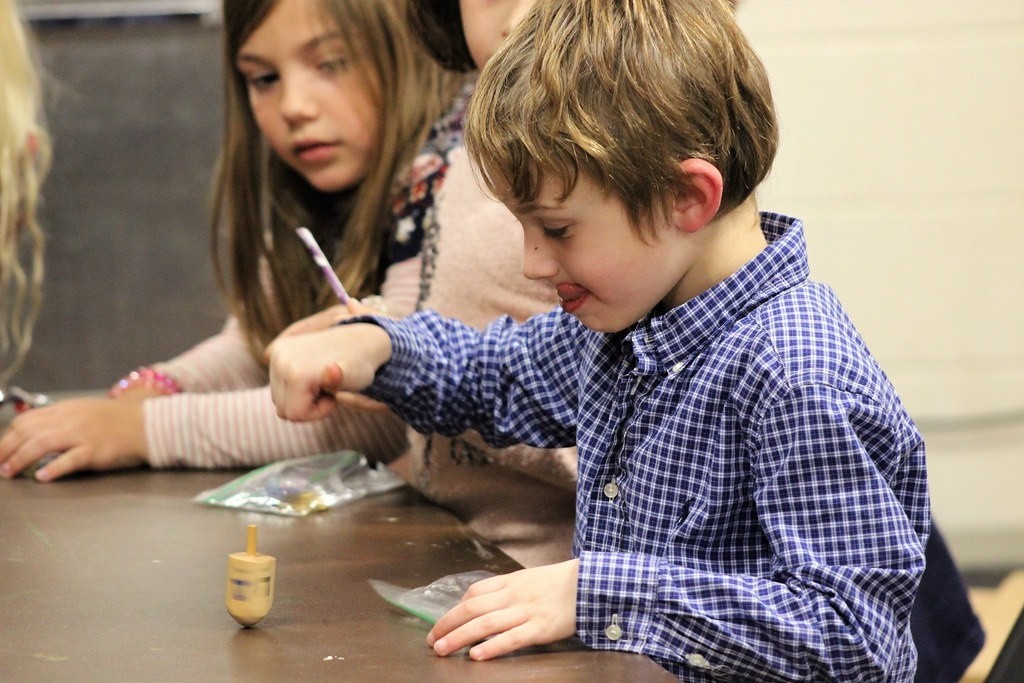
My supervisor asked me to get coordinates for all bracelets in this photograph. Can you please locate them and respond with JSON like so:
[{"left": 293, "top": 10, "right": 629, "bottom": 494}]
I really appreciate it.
[{"left": 109, "top": 365, "right": 180, "bottom": 395}]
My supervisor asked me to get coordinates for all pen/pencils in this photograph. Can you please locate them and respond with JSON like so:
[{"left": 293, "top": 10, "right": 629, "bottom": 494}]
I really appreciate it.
[
  {"left": 296, "top": 226, "right": 351, "bottom": 306},
  {"left": 9, "top": 390, "right": 34, "bottom": 415}
]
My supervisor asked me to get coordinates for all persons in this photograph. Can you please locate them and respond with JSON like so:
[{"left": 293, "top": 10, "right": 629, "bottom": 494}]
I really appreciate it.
[
  {"left": 269, "top": 2, "right": 932, "bottom": 683},
  {"left": 1, "top": 0, "right": 985, "bottom": 683}
]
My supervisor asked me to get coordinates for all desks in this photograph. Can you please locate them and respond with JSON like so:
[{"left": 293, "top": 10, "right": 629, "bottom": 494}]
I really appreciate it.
[{"left": 0, "top": 391, "right": 679, "bottom": 683}]
